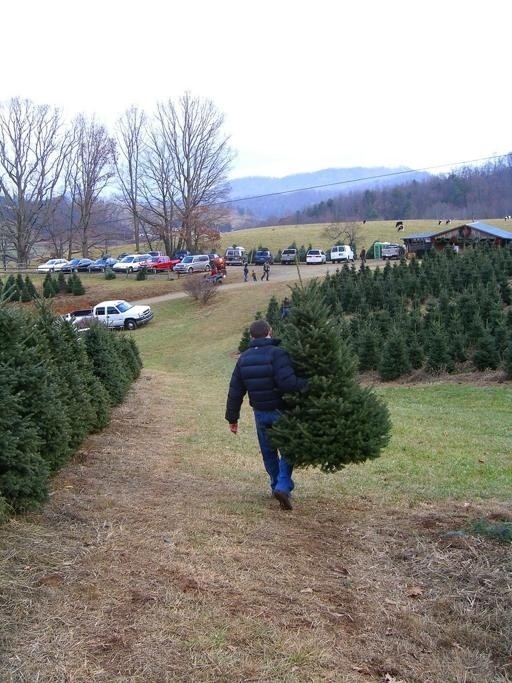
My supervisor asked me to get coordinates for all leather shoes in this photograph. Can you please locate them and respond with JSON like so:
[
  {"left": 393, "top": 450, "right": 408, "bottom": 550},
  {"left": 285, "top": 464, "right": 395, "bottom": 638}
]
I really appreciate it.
[{"left": 273, "top": 490, "right": 293, "bottom": 510}]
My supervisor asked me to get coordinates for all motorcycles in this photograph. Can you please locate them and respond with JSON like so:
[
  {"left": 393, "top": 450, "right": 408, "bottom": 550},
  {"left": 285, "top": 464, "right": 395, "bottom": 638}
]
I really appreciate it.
[{"left": 202, "top": 273, "right": 227, "bottom": 288}]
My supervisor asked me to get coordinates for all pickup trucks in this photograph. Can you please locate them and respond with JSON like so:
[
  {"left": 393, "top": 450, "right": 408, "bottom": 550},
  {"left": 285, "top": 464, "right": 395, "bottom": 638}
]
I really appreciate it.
[{"left": 63, "top": 298, "right": 156, "bottom": 333}]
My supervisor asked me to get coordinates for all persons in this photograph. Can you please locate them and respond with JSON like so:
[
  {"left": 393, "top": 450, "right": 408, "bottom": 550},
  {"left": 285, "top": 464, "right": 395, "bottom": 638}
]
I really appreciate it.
[
  {"left": 261, "top": 260, "right": 271, "bottom": 281},
  {"left": 359, "top": 245, "right": 366, "bottom": 265},
  {"left": 280, "top": 295, "right": 291, "bottom": 318},
  {"left": 252, "top": 269, "right": 257, "bottom": 281},
  {"left": 211, "top": 266, "right": 218, "bottom": 275},
  {"left": 243, "top": 262, "right": 249, "bottom": 281},
  {"left": 224, "top": 319, "right": 308, "bottom": 510},
  {"left": 212, "top": 272, "right": 224, "bottom": 284}
]
